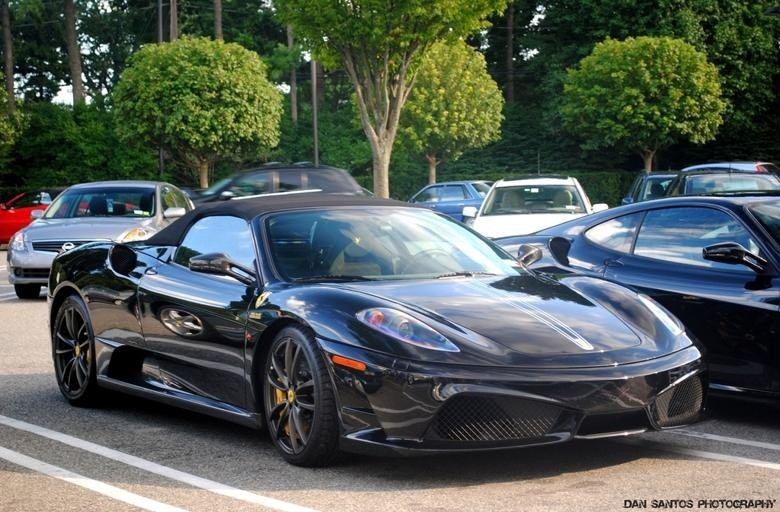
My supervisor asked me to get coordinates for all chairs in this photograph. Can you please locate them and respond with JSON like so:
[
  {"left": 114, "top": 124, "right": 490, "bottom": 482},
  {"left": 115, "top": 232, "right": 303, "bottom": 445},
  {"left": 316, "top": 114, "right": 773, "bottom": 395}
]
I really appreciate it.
[
  {"left": 646, "top": 182, "right": 665, "bottom": 198},
  {"left": 324, "top": 221, "right": 381, "bottom": 278},
  {"left": 552, "top": 190, "right": 573, "bottom": 208},
  {"left": 90, "top": 195, "right": 109, "bottom": 216},
  {"left": 138, "top": 193, "right": 153, "bottom": 215},
  {"left": 501, "top": 189, "right": 525, "bottom": 210}
]
